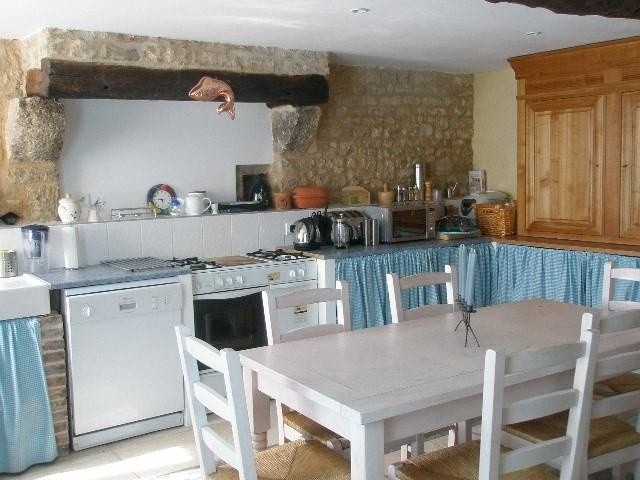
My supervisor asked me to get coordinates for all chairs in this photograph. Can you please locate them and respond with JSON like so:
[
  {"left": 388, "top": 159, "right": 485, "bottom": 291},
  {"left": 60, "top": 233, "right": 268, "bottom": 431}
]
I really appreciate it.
[
  {"left": 582, "top": 262, "right": 640, "bottom": 425},
  {"left": 174, "top": 323, "right": 351, "bottom": 478},
  {"left": 385, "top": 264, "right": 482, "bottom": 442},
  {"left": 495, "top": 307, "right": 638, "bottom": 478},
  {"left": 261, "top": 280, "right": 421, "bottom": 456},
  {"left": 387, "top": 329, "right": 600, "bottom": 478}
]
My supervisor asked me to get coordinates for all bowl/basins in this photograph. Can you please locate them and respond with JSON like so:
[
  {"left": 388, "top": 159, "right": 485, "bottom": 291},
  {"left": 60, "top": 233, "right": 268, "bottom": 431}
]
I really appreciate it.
[{"left": 378, "top": 191, "right": 396, "bottom": 203}]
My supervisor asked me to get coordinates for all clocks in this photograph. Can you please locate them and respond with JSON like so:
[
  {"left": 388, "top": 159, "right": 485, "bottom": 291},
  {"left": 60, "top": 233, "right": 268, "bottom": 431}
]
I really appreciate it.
[{"left": 147, "top": 184, "right": 177, "bottom": 215}]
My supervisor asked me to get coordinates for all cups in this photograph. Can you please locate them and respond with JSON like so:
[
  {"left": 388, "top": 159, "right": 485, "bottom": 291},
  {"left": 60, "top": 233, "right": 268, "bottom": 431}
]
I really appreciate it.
[
  {"left": 21, "top": 224, "right": 50, "bottom": 273},
  {"left": 273, "top": 192, "right": 290, "bottom": 211},
  {"left": 364, "top": 219, "right": 379, "bottom": 246}
]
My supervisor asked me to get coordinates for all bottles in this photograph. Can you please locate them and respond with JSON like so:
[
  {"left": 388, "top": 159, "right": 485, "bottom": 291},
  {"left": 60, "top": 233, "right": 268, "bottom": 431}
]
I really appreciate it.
[
  {"left": 396, "top": 185, "right": 420, "bottom": 202},
  {"left": 431, "top": 183, "right": 443, "bottom": 202},
  {"left": 334, "top": 218, "right": 350, "bottom": 249},
  {"left": 169, "top": 199, "right": 182, "bottom": 217},
  {"left": 57, "top": 193, "right": 82, "bottom": 223}
]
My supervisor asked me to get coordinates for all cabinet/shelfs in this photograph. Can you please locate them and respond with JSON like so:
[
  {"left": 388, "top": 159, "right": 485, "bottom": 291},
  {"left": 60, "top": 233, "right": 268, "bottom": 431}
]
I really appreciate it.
[{"left": 506, "top": 35, "right": 640, "bottom": 246}]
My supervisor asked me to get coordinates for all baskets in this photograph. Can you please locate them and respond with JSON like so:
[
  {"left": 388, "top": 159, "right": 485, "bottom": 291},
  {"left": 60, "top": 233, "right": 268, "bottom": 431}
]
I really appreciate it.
[{"left": 475, "top": 199, "right": 517, "bottom": 236}]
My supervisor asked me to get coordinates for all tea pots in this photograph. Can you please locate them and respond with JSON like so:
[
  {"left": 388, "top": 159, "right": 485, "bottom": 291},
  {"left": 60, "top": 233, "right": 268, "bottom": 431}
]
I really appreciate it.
[{"left": 178, "top": 192, "right": 211, "bottom": 216}]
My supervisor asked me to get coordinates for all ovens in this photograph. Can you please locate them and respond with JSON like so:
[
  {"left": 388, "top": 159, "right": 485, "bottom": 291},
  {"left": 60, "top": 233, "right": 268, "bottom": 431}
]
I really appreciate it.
[{"left": 189, "top": 280, "right": 320, "bottom": 416}]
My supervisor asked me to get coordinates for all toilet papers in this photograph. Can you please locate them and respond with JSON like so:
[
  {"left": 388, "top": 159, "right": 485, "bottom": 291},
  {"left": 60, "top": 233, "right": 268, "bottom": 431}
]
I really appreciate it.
[{"left": 61, "top": 227, "right": 82, "bottom": 269}]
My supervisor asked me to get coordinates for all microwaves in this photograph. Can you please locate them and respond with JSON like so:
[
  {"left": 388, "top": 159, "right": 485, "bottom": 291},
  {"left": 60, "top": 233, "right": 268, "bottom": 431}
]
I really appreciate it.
[{"left": 363, "top": 202, "right": 436, "bottom": 243}]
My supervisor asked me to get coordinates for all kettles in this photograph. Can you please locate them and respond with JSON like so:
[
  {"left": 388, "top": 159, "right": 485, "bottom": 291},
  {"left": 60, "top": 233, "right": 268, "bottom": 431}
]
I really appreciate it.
[{"left": 293, "top": 217, "right": 321, "bottom": 252}]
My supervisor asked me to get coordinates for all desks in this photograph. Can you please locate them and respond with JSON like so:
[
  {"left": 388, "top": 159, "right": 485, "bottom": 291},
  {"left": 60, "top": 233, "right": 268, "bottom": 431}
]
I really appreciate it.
[{"left": 230, "top": 297, "right": 640, "bottom": 480}]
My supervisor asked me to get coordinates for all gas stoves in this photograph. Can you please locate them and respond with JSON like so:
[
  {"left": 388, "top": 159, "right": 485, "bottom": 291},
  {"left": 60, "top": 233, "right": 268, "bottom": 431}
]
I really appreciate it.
[{"left": 169, "top": 249, "right": 319, "bottom": 296}]
